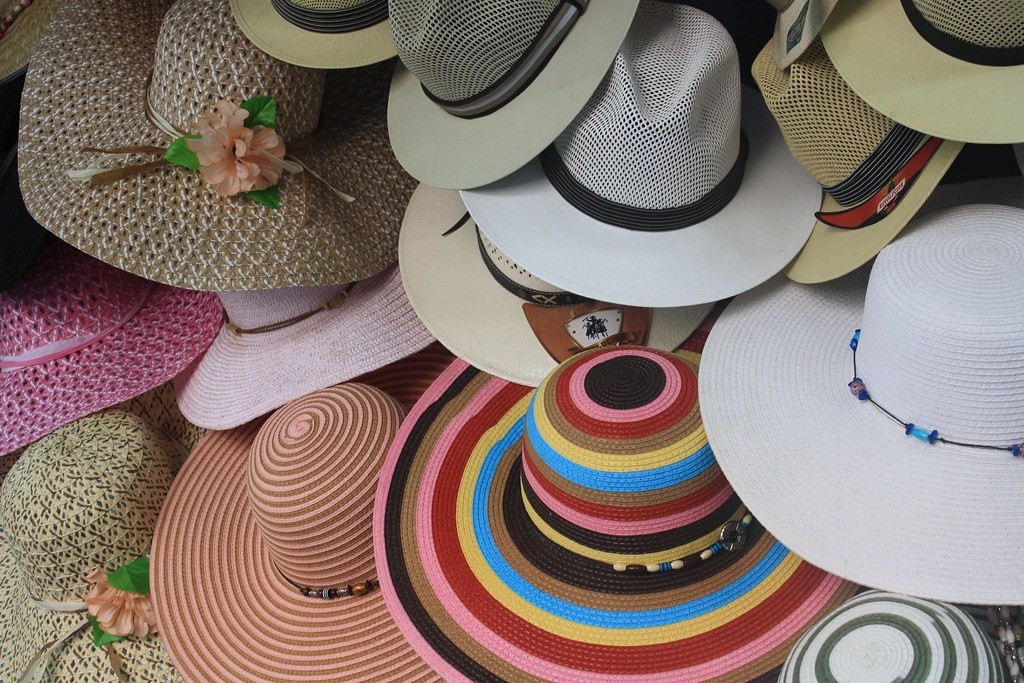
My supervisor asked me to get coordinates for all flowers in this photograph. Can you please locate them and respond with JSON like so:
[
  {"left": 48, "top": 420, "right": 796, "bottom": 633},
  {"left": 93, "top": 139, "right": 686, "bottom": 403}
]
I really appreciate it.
[
  {"left": 85, "top": 551, "right": 160, "bottom": 646},
  {"left": 168, "top": 93, "right": 304, "bottom": 212}
]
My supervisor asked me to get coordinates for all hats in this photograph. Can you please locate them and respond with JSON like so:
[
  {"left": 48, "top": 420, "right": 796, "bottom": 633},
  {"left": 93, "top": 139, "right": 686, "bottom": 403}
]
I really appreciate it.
[{"left": 0, "top": 0, "right": 1024, "bottom": 683}]
